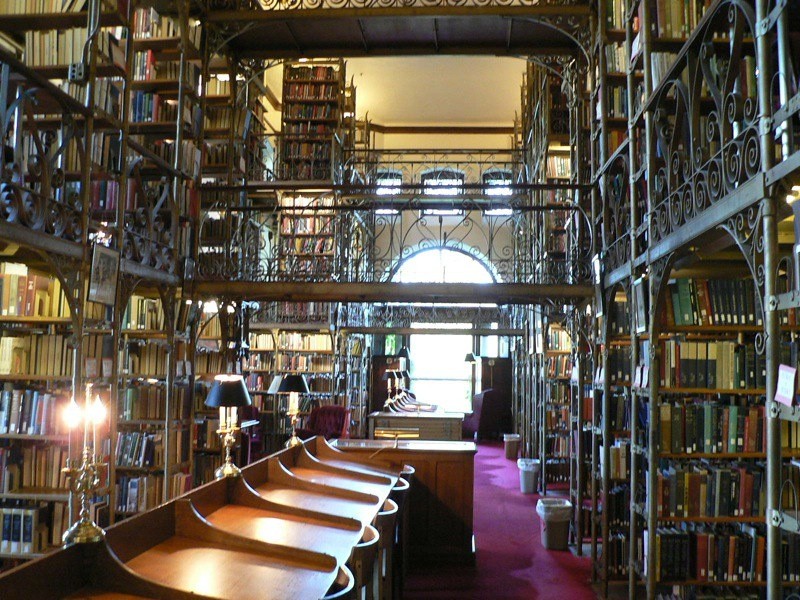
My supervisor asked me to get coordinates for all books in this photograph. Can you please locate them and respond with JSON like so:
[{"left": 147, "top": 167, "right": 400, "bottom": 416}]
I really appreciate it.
[
  {"left": 512, "top": 0, "right": 800, "bottom": 600},
  {"left": 0, "top": 0, "right": 341, "bottom": 561}
]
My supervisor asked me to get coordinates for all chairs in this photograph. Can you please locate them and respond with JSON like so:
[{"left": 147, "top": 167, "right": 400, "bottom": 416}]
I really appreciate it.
[
  {"left": 296, "top": 405, "right": 349, "bottom": 440},
  {"left": 320, "top": 464, "right": 416, "bottom": 600}
]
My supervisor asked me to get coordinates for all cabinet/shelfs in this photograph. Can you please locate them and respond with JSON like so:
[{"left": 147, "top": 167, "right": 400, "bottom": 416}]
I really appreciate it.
[
  {"left": 511, "top": 0, "right": 799, "bottom": 600},
  {"left": 0, "top": 0, "right": 375, "bottom": 560}
]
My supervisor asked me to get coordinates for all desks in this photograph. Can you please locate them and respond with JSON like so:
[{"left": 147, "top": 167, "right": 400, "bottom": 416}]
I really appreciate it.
[
  {"left": 0, "top": 440, "right": 404, "bottom": 600},
  {"left": 328, "top": 439, "right": 477, "bottom": 562},
  {"left": 367, "top": 411, "right": 465, "bottom": 440}
]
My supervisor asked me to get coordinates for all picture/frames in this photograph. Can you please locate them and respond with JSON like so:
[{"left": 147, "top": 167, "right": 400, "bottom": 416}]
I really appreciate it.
[{"left": 86, "top": 243, "right": 121, "bottom": 306}]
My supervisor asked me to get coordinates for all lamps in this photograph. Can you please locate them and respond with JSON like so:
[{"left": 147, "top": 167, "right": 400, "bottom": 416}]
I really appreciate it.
[
  {"left": 374, "top": 428, "right": 419, "bottom": 448},
  {"left": 464, "top": 353, "right": 476, "bottom": 362},
  {"left": 397, "top": 347, "right": 410, "bottom": 359},
  {"left": 60, "top": 384, "right": 108, "bottom": 545},
  {"left": 204, "top": 374, "right": 252, "bottom": 478},
  {"left": 267, "top": 376, "right": 310, "bottom": 448},
  {"left": 382, "top": 369, "right": 409, "bottom": 404}
]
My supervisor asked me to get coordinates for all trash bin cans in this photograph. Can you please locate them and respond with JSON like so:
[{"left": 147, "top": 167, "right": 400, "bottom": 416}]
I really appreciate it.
[
  {"left": 517, "top": 459, "right": 541, "bottom": 493},
  {"left": 503, "top": 434, "right": 521, "bottom": 459},
  {"left": 536, "top": 498, "right": 573, "bottom": 550}
]
